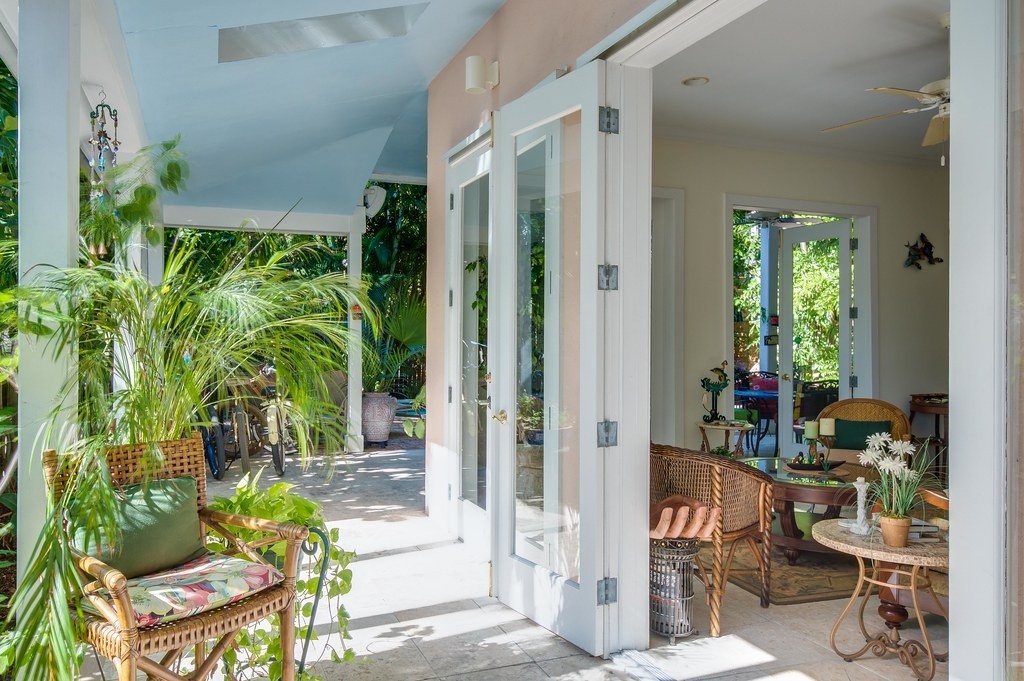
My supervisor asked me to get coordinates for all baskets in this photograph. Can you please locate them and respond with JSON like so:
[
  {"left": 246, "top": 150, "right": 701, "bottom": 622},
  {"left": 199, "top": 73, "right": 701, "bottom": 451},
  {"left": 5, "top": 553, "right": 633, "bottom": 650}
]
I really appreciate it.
[{"left": 249, "top": 371, "right": 279, "bottom": 400}]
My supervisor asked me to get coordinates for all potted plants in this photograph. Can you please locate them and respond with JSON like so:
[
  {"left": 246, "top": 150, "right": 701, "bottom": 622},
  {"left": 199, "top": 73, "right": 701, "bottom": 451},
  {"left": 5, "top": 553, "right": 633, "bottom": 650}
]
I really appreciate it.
[{"left": 341, "top": 336, "right": 426, "bottom": 440}]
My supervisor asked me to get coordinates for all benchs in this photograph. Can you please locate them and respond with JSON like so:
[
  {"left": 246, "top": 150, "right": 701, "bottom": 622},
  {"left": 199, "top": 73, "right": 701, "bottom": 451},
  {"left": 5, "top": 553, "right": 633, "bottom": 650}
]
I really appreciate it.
[
  {"left": 736, "top": 372, "right": 807, "bottom": 450},
  {"left": 794, "top": 379, "right": 854, "bottom": 445}
]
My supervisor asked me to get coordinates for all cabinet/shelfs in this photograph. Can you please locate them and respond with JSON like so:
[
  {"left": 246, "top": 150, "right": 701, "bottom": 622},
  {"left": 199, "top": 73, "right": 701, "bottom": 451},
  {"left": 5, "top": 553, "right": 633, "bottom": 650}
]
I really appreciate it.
[{"left": 909, "top": 393, "right": 949, "bottom": 489}]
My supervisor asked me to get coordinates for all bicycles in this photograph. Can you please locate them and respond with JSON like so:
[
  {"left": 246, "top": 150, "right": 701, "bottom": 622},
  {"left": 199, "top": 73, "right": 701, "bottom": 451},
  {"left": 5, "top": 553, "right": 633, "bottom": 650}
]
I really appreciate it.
[{"left": 194, "top": 363, "right": 311, "bottom": 481}]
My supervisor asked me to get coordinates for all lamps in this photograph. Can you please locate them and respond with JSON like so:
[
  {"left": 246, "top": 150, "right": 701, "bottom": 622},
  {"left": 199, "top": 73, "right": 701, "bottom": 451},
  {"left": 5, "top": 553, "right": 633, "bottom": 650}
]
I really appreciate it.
[
  {"left": 919, "top": 104, "right": 950, "bottom": 167},
  {"left": 466, "top": 55, "right": 500, "bottom": 96}
]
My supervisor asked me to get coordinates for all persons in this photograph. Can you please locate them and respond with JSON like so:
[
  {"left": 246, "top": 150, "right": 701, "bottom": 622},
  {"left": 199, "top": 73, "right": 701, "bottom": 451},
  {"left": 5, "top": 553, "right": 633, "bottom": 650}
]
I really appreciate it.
[{"left": 650, "top": 495, "right": 721, "bottom": 540}]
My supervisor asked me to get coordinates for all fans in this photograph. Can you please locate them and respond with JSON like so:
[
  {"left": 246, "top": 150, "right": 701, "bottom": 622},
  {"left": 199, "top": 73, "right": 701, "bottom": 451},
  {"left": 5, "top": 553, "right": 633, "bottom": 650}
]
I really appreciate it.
[{"left": 820, "top": 76, "right": 950, "bottom": 134}]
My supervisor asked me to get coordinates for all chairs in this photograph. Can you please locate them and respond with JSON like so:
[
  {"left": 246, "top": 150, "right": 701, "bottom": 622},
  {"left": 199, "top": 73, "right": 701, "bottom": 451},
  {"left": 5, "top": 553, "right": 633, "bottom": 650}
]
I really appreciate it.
[
  {"left": 42, "top": 431, "right": 310, "bottom": 681},
  {"left": 816, "top": 398, "right": 911, "bottom": 483},
  {"left": 650, "top": 441, "right": 776, "bottom": 638}
]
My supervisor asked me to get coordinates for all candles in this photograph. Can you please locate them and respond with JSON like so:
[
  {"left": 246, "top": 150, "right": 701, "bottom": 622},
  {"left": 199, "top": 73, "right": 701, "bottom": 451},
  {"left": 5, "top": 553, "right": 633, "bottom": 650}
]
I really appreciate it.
[{"left": 856, "top": 476, "right": 866, "bottom": 484}]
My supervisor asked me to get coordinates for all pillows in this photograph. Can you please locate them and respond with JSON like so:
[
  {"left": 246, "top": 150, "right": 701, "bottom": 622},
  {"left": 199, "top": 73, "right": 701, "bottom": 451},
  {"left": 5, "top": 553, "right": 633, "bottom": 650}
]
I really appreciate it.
[{"left": 60, "top": 475, "right": 211, "bottom": 582}]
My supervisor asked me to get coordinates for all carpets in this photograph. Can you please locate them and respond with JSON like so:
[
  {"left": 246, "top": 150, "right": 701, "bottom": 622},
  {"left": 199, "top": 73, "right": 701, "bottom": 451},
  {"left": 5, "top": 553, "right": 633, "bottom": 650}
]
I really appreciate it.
[{"left": 693, "top": 541, "right": 880, "bottom": 605}]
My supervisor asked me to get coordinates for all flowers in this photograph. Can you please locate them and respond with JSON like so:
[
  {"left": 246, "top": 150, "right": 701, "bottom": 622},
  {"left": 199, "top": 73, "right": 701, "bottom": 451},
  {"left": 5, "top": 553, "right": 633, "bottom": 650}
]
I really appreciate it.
[
  {"left": 518, "top": 387, "right": 546, "bottom": 442},
  {"left": 838, "top": 431, "right": 949, "bottom": 574}
]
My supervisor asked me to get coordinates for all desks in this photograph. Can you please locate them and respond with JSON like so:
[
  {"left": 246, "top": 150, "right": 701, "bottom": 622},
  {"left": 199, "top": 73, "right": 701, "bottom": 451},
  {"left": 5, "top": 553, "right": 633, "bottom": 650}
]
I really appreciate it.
[
  {"left": 697, "top": 421, "right": 755, "bottom": 459},
  {"left": 811, "top": 516, "right": 948, "bottom": 681},
  {"left": 738, "top": 457, "right": 893, "bottom": 565},
  {"left": 734, "top": 389, "right": 799, "bottom": 458}
]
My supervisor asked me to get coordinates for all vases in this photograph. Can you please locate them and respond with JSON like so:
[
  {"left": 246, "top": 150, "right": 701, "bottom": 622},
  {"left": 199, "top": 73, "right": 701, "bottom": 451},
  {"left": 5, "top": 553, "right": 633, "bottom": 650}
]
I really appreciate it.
[
  {"left": 526, "top": 428, "right": 544, "bottom": 445},
  {"left": 882, "top": 514, "right": 912, "bottom": 547}
]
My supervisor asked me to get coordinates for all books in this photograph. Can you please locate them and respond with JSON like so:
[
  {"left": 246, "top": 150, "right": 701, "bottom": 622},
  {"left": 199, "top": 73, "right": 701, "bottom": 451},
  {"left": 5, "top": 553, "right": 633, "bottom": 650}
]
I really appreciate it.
[{"left": 873, "top": 513, "right": 941, "bottom": 543}]
